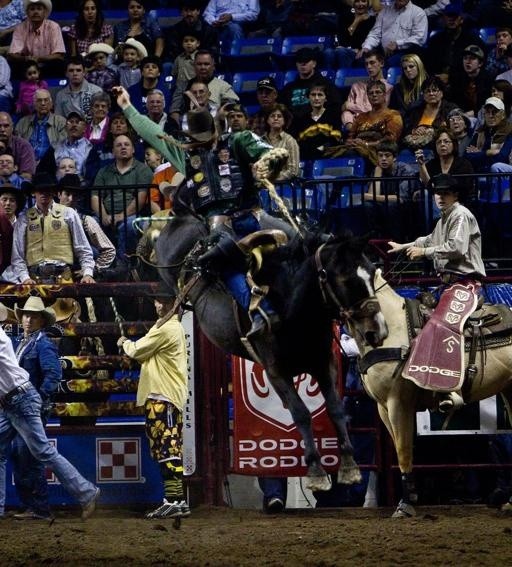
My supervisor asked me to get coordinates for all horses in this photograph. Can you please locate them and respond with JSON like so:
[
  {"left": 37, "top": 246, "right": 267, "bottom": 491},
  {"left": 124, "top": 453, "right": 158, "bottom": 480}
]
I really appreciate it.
[
  {"left": 129, "top": 207, "right": 389, "bottom": 491},
  {"left": 346, "top": 266, "right": 512, "bottom": 519}
]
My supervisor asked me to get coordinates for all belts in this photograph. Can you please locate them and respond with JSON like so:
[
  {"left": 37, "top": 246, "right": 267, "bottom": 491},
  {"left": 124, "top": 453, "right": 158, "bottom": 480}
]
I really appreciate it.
[{"left": 7, "top": 381, "right": 32, "bottom": 396}]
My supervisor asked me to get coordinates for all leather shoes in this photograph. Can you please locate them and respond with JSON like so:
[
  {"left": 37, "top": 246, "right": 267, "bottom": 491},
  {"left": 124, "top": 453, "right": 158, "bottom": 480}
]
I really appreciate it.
[
  {"left": 14, "top": 511, "right": 51, "bottom": 520},
  {"left": 82, "top": 487, "right": 100, "bottom": 519}
]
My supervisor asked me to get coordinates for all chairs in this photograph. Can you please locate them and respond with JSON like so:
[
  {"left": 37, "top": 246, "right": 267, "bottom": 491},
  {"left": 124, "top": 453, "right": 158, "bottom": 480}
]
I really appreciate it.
[{"left": 0, "top": 0, "right": 512, "bottom": 229}]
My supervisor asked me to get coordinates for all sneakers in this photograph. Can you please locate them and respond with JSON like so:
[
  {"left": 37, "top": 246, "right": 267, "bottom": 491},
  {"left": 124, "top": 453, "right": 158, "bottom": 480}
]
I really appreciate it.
[
  {"left": 246, "top": 314, "right": 280, "bottom": 337},
  {"left": 145, "top": 498, "right": 191, "bottom": 518}
]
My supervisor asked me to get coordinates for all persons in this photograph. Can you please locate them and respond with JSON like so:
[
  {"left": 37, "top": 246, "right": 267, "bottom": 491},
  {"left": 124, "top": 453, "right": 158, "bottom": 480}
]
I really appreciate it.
[
  {"left": 0, "top": 302, "right": 102, "bottom": 524},
  {"left": 385, "top": 171, "right": 486, "bottom": 413},
  {"left": 0, "top": 0, "right": 512, "bottom": 320},
  {"left": 114, "top": 84, "right": 291, "bottom": 339},
  {"left": 117, "top": 281, "right": 194, "bottom": 518},
  {"left": 11, "top": 293, "right": 66, "bottom": 522}
]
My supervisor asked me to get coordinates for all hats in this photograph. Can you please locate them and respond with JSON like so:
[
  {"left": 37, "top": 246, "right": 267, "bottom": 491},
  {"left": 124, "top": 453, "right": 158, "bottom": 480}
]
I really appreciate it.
[
  {"left": 0, "top": 145, "right": 85, "bottom": 211},
  {"left": 14, "top": 296, "right": 56, "bottom": 325},
  {"left": 257, "top": 77, "right": 277, "bottom": 90},
  {"left": 484, "top": 97, "right": 505, "bottom": 110},
  {"left": 179, "top": 108, "right": 215, "bottom": 142},
  {"left": 431, "top": 174, "right": 457, "bottom": 188},
  {"left": 80, "top": 38, "right": 148, "bottom": 58}
]
